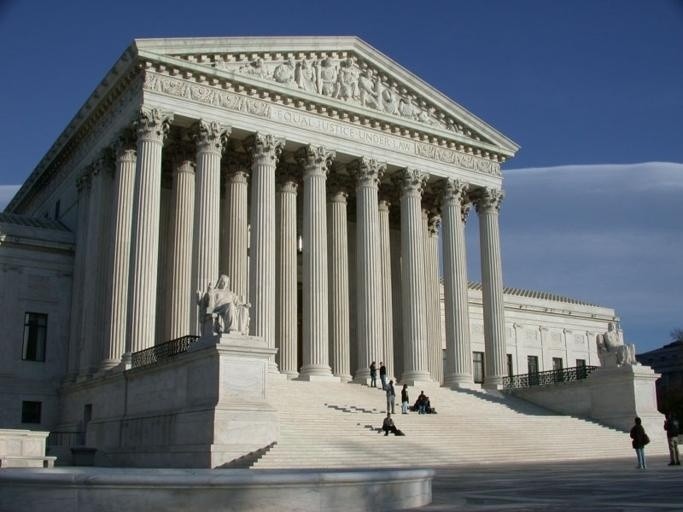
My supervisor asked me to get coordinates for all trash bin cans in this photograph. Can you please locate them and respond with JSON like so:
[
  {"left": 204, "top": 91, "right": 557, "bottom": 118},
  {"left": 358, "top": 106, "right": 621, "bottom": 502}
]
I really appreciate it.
[{"left": 70, "top": 448, "right": 97, "bottom": 466}]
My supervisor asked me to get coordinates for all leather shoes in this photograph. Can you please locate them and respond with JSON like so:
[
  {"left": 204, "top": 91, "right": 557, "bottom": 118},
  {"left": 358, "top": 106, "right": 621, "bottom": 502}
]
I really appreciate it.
[
  {"left": 668, "top": 461, "right": 675, "bottom": 465},
  {"left": 675, "top": 461, "right": 680, "bottom": 465}
]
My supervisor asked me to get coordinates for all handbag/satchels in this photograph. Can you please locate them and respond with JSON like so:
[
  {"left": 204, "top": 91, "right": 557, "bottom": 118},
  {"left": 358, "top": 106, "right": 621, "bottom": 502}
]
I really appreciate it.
[{"left": 643, "top": 434, "right": 649, "bottom": 444}]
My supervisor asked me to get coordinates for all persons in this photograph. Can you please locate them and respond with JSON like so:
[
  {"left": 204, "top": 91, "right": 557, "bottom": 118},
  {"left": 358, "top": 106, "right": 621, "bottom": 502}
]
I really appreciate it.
[
  {"left": 368, "top": 361, "right": 377, "bottom": 388},
  {"left": 399, "top": 383, "right": 409, "bottom": 414},
  {"left": 663, "top": 411, "right": 681, "bottom": 467},
  {"left": 412, "top": 390, "right": 436, "bottom": 414},
  {"left": 382, "top": 413, "right": 399, "bottom": 436},
  {"left": 204, "top": 273, "right": 253, "bottom": 336},
  {"left": 385, "top": 379, "right": 396, "bottom": 414},
  {"left": 378, "top": 360, "right": 387, "bottom": 390},
  {"left": 603, "top": 322, "right": 638, "bottom": 367},
  {"left": 629, "top": 416, "right": 650, "bottom": 470},
  {"left": 239, "top": 52, "right": 435, "bottom": 127}
]
